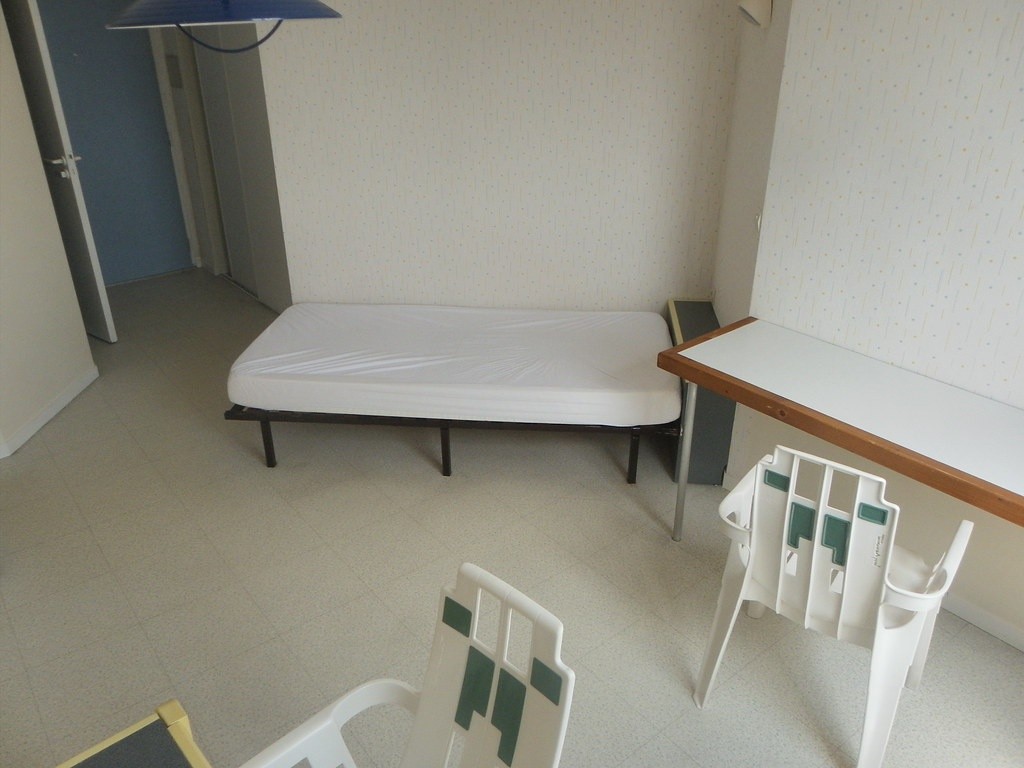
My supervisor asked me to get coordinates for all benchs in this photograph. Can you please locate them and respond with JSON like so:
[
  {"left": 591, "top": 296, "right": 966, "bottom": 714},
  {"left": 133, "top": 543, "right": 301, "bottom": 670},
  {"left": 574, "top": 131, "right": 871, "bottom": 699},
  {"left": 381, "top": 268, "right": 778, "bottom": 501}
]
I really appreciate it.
[{"left": 221, "top": 303, "right": 680, "bottom": 485}]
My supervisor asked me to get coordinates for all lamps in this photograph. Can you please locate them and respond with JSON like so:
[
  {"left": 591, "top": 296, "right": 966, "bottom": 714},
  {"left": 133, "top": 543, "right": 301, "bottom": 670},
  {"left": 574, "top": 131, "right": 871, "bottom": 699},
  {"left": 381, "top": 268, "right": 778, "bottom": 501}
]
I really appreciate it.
[
  {"left": 105, "top": 0, "right": 341, "bottom": 54},
  {"left": 737, "top": 0, "right": 772, "bottom": 28}
]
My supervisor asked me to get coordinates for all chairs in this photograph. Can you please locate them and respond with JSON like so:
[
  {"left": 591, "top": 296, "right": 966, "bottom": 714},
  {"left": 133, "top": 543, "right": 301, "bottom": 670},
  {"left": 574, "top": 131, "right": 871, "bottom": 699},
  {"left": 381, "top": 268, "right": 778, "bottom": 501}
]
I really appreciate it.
[
  {"left": 691, "top": 442, "right": 974, "bottom": 768},
  {"left": 237, "top": 562, "right": 578, "bottom": 768}
]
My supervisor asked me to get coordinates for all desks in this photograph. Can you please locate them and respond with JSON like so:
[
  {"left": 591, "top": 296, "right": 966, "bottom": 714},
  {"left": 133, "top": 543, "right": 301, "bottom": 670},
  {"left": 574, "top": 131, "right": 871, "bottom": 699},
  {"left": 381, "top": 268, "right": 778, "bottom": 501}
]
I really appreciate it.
[
  {"left": 55, "top": 700, "right": 213, "bottom": 768},
  {"left": 657, "top": 317, "right": 1024, "bottom": 544}
]
